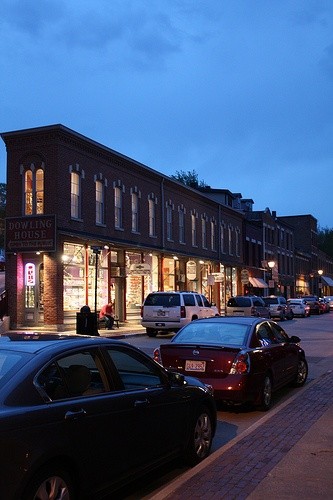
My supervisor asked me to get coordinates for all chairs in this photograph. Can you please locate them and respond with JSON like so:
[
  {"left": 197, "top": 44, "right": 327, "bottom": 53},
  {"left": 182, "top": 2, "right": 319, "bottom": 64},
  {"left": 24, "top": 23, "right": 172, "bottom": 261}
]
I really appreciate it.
[{"left": 64, "top": 365, "right": 91, "bottom": 398}]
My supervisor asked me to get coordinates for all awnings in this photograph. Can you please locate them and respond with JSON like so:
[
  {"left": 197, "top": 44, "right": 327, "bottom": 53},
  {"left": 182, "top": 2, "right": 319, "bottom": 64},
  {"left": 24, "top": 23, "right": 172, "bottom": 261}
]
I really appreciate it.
[
  {"left": 248, "top": 276, "right": 268, "bottom": 288},
  {"left": 321, "top": 276, "right": 333, "bottom": 286}
]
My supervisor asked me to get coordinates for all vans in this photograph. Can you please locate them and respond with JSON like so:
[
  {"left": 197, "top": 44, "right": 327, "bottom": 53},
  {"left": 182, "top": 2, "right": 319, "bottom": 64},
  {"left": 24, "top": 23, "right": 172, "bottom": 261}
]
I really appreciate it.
[
  {"left": 141, "top": 290, "right": 221, "bottom": 338},
  {"left": 259, "top": 294, "right": 293, "bottom": 322},
  {"left": 226, "top": 294, "right": 271, "bottom": 319}
]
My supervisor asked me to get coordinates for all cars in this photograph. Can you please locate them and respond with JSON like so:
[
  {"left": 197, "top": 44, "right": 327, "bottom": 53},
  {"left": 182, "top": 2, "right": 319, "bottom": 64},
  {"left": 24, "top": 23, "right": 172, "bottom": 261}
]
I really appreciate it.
[
  {"left": 286, "top": 298, "right": 311, "bottom": 318},
  {"left": 152, "top": 315, "right": 308, "bottom": 411},
  {"left": 301, "top": 295, "right": 333, "bottom": 315},
  {"left": 0, "top": 330, "right": 218, "bottom": 500}
]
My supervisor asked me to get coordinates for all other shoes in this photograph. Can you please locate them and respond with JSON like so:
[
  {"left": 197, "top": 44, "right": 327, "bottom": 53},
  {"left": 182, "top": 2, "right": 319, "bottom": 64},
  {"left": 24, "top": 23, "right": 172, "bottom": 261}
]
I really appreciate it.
[{"left": 105, "top": 327, "right": 115, "bottom": 330}]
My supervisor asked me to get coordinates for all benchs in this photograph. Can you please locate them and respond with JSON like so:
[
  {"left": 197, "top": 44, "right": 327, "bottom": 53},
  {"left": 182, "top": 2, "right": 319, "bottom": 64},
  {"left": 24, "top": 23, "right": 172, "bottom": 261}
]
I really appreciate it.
[{"left": 97, "top": 313, "right": 120, "bottom": 330}]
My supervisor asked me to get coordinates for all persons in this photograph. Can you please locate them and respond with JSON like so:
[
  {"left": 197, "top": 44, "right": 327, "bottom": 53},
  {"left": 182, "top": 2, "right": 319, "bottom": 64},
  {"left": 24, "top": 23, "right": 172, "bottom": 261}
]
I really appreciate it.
[{"left": 99, "top": 302, "right": 117, "bottom": 330}]
